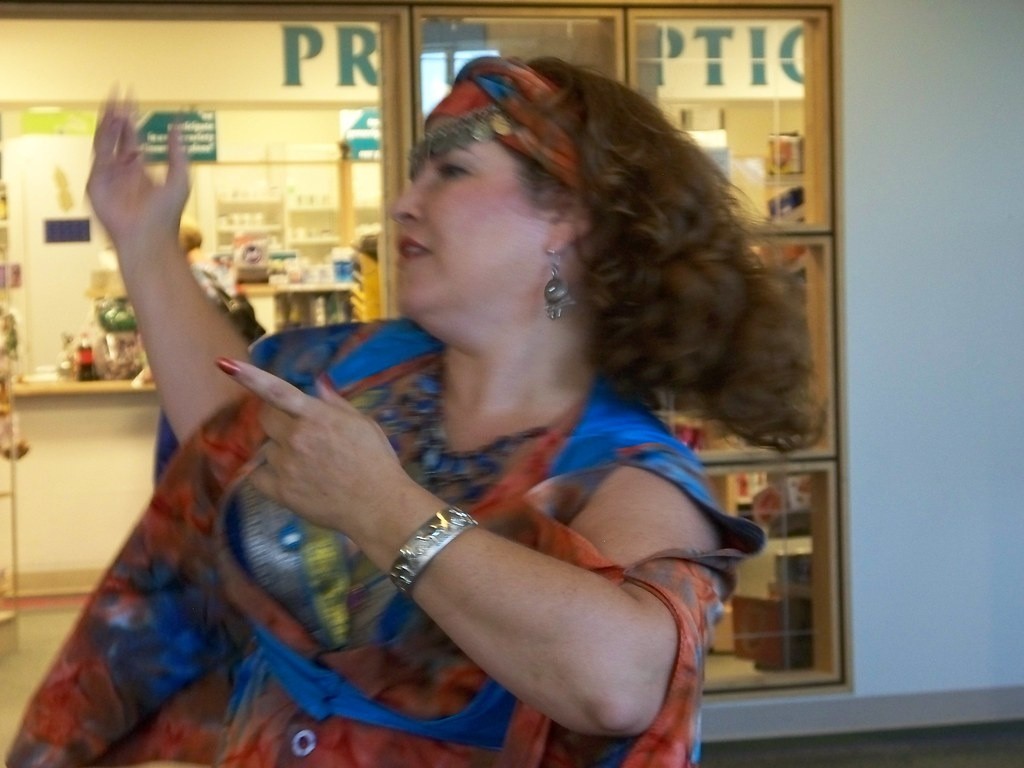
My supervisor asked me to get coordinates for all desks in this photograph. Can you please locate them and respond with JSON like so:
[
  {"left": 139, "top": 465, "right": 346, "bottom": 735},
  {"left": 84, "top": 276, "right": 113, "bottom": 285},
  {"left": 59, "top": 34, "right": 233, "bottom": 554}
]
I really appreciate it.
[
  {"left": 0, "top": 382, "right": 161, "bottom": 599},
  {"left": 235, "top": 284, "right": 353, "bottom": 332}
]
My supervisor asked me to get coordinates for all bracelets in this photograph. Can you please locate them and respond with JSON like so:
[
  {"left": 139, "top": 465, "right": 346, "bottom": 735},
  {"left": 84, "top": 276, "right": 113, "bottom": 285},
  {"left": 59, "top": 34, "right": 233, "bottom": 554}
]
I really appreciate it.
[{"left": 391, "top": 503, "right": 480, "bottom": 601}]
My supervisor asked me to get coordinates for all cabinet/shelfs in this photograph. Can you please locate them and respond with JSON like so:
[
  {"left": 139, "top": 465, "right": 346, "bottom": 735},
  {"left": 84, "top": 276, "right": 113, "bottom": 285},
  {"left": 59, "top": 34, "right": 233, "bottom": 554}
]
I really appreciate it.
[{"left": 195, "top": 163, "right": 381, "bottom": 265}]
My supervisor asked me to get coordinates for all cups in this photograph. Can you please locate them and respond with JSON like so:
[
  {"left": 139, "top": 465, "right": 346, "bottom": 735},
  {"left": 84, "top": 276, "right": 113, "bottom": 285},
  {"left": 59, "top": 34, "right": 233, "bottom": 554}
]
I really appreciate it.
[{"left": 332, "top": 247, "right": 354, "bottom": 281}]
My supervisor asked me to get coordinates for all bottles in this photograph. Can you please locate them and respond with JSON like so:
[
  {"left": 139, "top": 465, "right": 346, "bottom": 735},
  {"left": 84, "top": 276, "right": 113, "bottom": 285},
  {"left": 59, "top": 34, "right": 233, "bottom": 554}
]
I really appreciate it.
[{"left": 77, "top": 331, "right": 93, "bottom": 381}]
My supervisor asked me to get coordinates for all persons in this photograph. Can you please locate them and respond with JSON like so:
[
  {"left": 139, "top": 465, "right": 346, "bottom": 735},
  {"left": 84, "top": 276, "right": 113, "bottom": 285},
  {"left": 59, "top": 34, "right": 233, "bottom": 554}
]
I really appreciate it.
[{"left": 9, "top": 52, "right": 815, "bottom": 768}]
